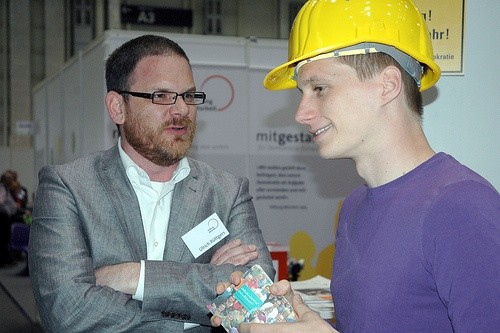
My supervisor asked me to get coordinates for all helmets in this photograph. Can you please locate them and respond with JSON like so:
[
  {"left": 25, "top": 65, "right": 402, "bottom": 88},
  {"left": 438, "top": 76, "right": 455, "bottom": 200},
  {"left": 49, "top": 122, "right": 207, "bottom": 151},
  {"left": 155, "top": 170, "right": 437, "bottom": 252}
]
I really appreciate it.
[{"left": 263, "top": 0, "right": 441, "bottom": 93}]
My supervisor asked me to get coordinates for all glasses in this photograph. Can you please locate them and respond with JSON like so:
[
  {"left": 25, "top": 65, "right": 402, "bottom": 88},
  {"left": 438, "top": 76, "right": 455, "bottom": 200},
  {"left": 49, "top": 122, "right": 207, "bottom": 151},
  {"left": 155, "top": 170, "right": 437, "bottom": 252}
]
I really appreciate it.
[{"left": 118, "top": 88, "right": 207, "bottom": 106}]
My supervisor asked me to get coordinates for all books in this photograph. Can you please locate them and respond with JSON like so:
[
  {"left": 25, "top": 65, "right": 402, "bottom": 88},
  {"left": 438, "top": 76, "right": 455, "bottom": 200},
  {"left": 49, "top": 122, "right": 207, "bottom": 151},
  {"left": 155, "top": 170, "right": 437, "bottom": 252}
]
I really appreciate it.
[{"left": 284, "top": 274, "right": 338, "bottom": 320}]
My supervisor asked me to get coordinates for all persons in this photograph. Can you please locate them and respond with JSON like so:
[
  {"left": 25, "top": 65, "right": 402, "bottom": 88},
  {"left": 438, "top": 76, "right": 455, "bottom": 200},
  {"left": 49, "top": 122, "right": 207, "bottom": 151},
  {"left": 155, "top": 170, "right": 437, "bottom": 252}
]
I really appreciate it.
[
  {"left": 210, "top": 0, "right": 500, "bottom": 333},
  {"left": 27, "top": 34, "right": 277, "bottom": 333},
  {"left": 0, "top": 170, "right": 32, "bottom": 276}
]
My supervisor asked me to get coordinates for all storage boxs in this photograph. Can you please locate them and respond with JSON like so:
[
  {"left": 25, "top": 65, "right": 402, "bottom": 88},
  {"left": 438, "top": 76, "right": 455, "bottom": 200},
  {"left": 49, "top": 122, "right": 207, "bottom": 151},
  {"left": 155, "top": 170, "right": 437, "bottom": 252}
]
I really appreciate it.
[{"left": 266, "top": 242, "right": 290, "bottom": 284}]
